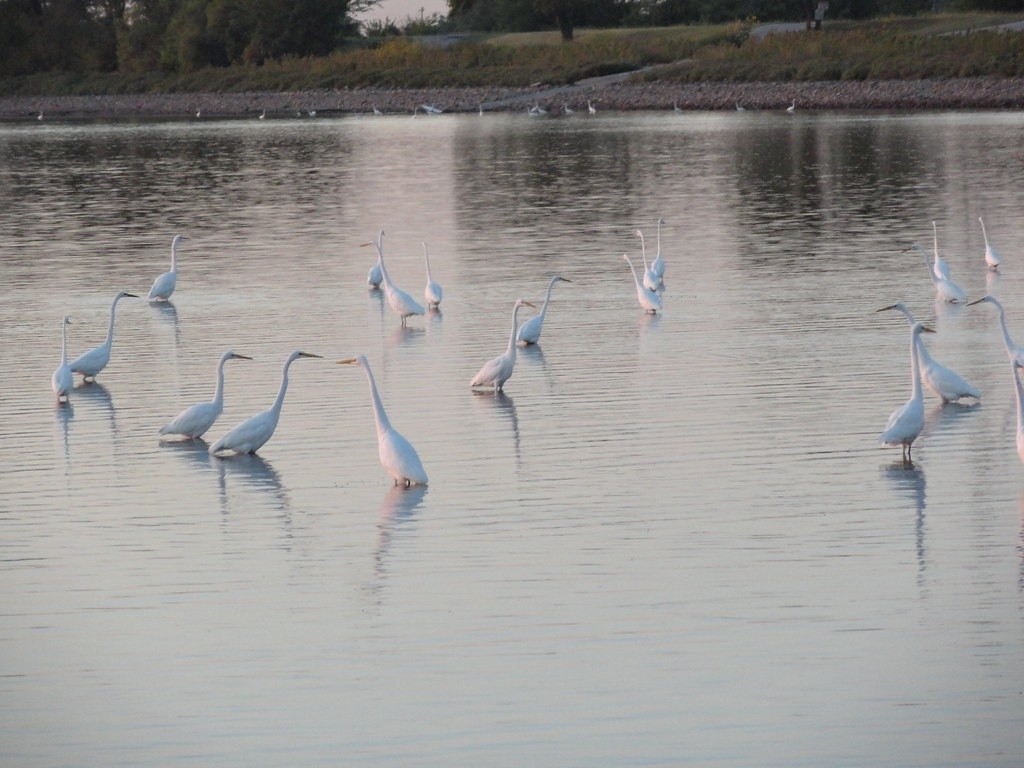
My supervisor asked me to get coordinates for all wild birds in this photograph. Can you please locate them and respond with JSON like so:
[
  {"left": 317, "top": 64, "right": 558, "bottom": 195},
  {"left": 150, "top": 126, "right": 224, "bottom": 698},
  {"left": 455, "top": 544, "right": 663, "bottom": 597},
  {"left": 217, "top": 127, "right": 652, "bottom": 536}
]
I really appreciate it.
[
  {"left": 207, "top": 349, "right": 324, "bottom": 455},
  {"left": 931, "top": 221, "right": 950, "bottom": 281},
  {"left": 359, "top": 239, "right": 424, "bottom": 328},
  {"left": 900, "top": 243, "right": 965, "bottom": 303},
  {"left": 194, "top": 96, "right": 799, "bottom": 121},
  {"left": 623, "top": 253, "right": 663, "bottom": 314},
  {"left": 159, "top": 350, "right": 253, "bottom": 439},
  {"left": 979, "top": 217, "right": 1000, "bottom": 271},
  {"left": 421, "top": 241, "right": 442, "bottom": 311},
  {"left": 334, "top": 354, "right": 429, "bottom": 491},
  {"left": 148, "top": 235, "right": 190, "bottom": 302},
  {"left": 36, "top": 110, "right": 45, "bottom": 121},
  {"left": 469, "top": 299, "right": 537, "bottom": 402},
  {"left": 367, "top": 229, "right": 386, "bottom": 289},
  {"left": 650, "top": 217, "right": 666, "bottom": 284},
  {"left": 876, "top": 322, "right": 937, "bottom": 466},
  {"left": 966, "top": 296, "right": 1024, "bottom": 369},
  {"left": 635, "top": 229, "right": 659, "bottom": 293},
  {"left": 52, "top": 316, "right": 74, "bottom": 405},
  {"left": 1011, "top": 359, "right": 1024, "bottom": 461},
  {"left": 68, "top": 291, "right": 140, "bottom": 384},
  {"left": 512, "top": 275, "right": 574, "bottom": 345},
  {"left": 876, "top": 302, "right": 982, "bottom": 405}
]
